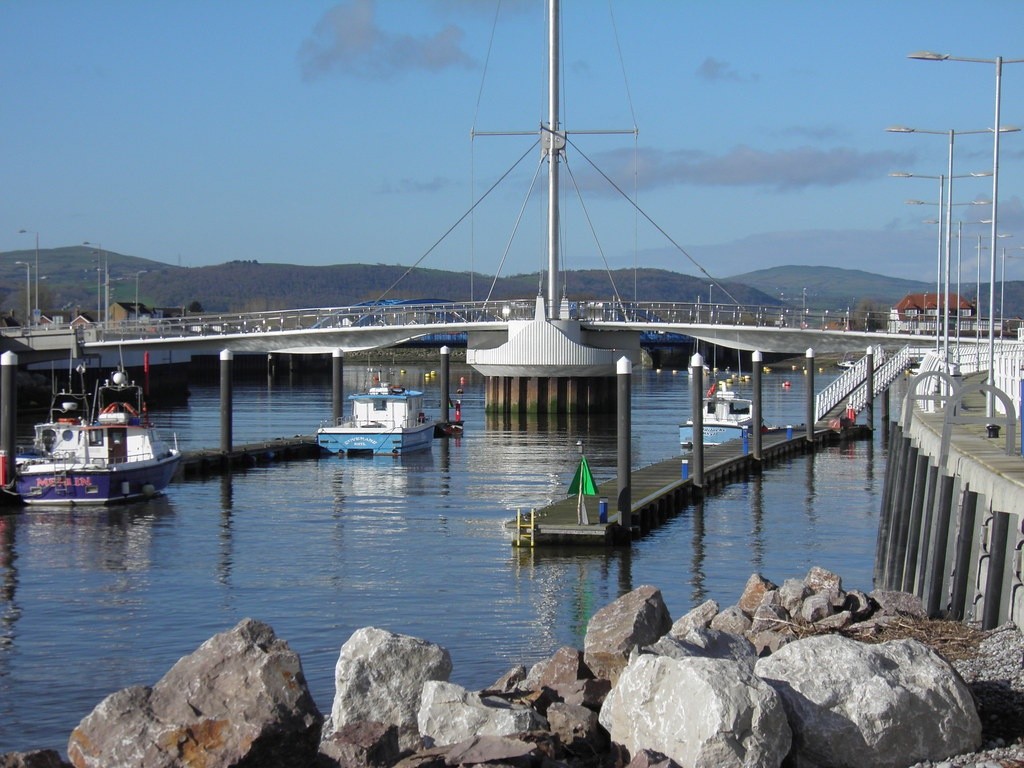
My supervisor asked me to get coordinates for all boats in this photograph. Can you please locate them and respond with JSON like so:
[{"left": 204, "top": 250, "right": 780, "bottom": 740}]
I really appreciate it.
[
  {"left": 15, "top": 324, "right": 184, "bottom": 505},
  {"left": 836, "top": 360, "right": 857, "bottom": 370},
  {"left": 678, "top": 326, "right": 764, "bottom": 449},
  {"left": 315, "top": 381, "right": 436, "bottom": 456}
]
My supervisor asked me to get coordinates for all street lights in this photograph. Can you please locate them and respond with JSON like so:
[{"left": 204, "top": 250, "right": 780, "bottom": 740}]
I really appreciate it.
[
  {"left": 888, "top": 168, "right": 994, "bottom": 394},
  {"left": 886, "top": 124, "right": 1022, "bottom": 409},
  {"left": 709, "top": 284, "right": 714, "bottom": 311},
  {"left": 906, "top": 50, "right": 1024, "bottom": 439},
  {"left": 922, "top": 218, "right": 998, "bottom": 364},
  {"left": 974, "top": 245, "right": 1023, "bottom": 357},
  {"left": 92, "top": 250, "right": 110, "bottom": 329},
  {"left": 82, "top": 241, "right": 102, "bottom": 324},
  {"left": 951, "top": 232, "right": 1015, "bottom": 373},
  {"left": 924, "top": 291, "right": 929, "bottom": 322},
  {"left": 16, "top": 261, "right": 31, "bottom": 326},
  {"left": 135, "top": 270, "right": 147, "bottom": 329},
  {"left": 18, "top": 230, "right": 39, "bottom": 327}
]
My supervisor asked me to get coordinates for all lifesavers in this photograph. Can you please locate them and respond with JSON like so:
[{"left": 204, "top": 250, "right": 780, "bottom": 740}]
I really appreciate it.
[
  {"left": 57, "top": 417, "right": 78, "bottom": 424},
  {"left": 101, "top": 402, "right": 118, "bottom": 416},
  {"left": 391, "top": 385, "right": 405, "bottom": 394},
  {"left": 123, "top": 402, "right": 138, "bottom": 417}
]
